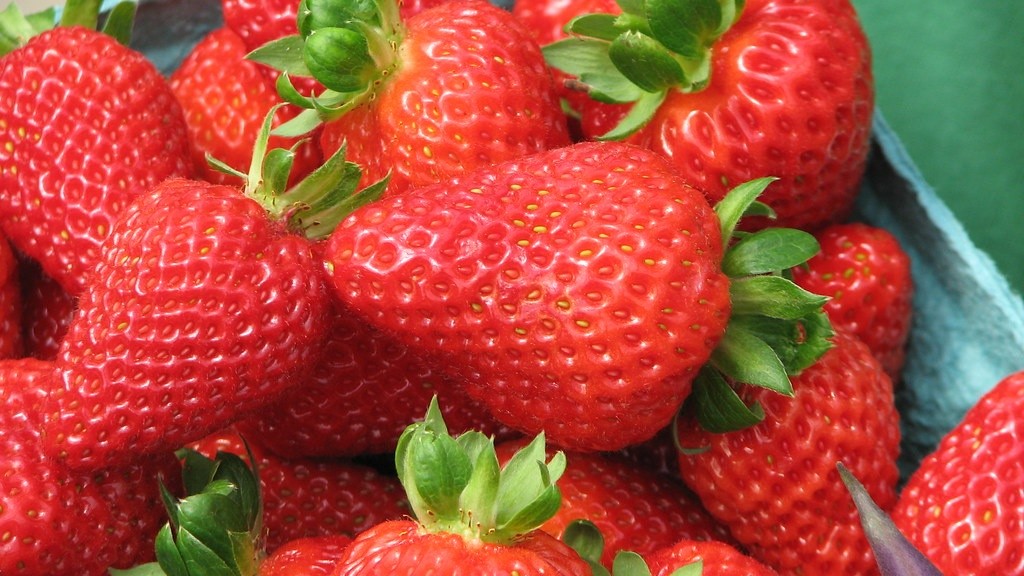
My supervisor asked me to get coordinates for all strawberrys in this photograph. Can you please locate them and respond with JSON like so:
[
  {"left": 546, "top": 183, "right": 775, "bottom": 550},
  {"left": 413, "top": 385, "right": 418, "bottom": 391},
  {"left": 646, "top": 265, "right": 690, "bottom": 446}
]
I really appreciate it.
[{"left": 0, "top": 0, "right": 1024, "bottom": 575}]
